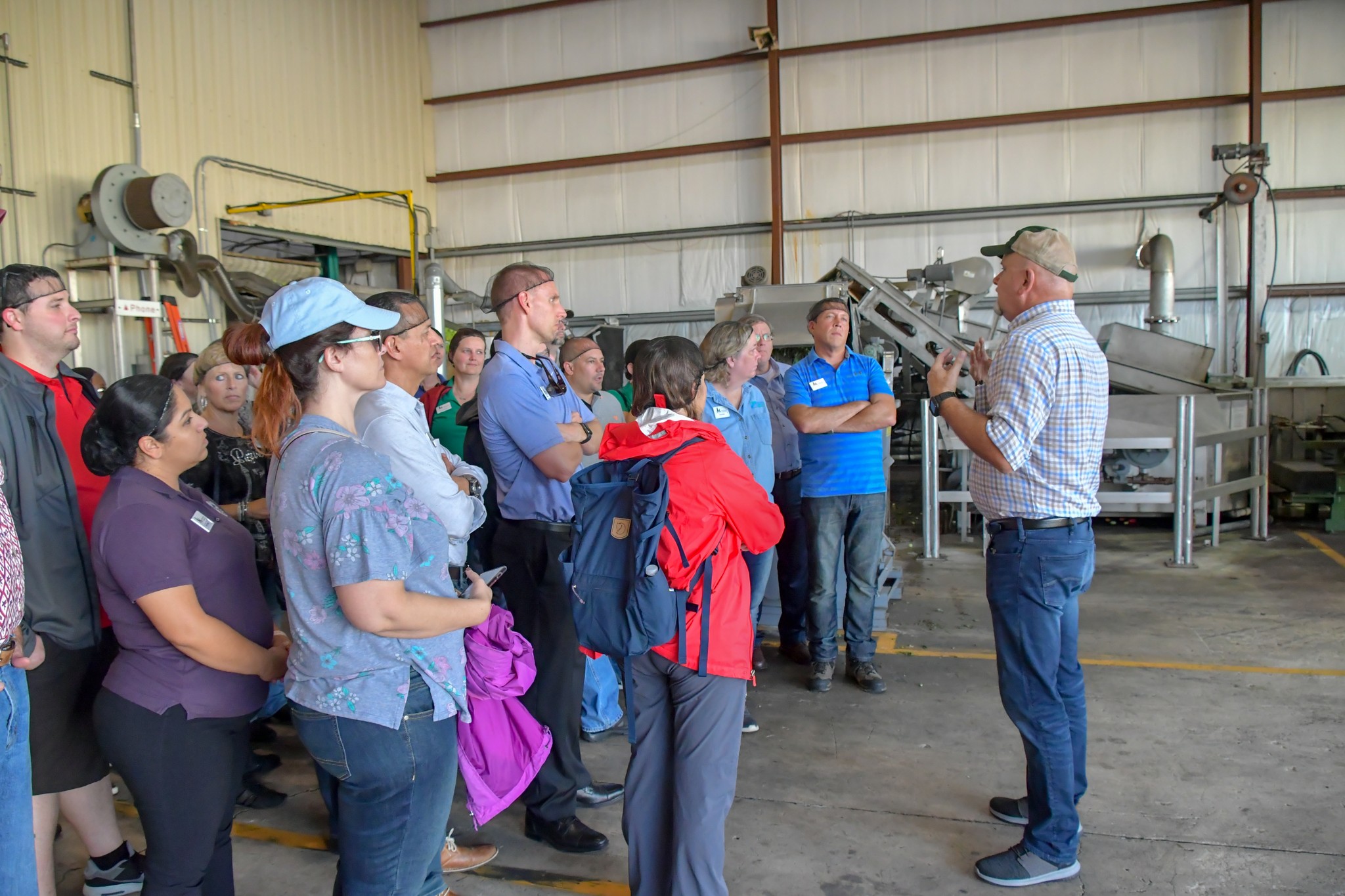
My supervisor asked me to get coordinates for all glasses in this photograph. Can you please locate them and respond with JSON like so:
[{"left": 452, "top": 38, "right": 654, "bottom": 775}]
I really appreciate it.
[
  {"left": 518, "top": 353, "right": 567, "bottom": 397},
  {"left": 96, "top": 386, "right": 107, "bottom": 395},
  {"left": 754, "top": 333, "right": 774, "bottom": 342},
  {"left": 317, "top": 331, "right": 383, "bottom": 363}
]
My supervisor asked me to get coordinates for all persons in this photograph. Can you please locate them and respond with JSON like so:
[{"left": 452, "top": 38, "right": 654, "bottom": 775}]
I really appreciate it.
[
  {"left": 924, "top": 225, "right": 1111, "bottom": 890},
  {"left": 475, "top": 259, "right": 627, "bottom": 855},
  {"left": 413, "top": 326, "right": 449, "bottom": 400},
  {"left": 177, "top": 338, "right": 292, "bottom": 811},
  {"left": 0, "top": 463, "right": 46, "bottom": 895},
  {"left": 158, "top": 352, "right": 262, "bottom": 430},
  {"left": 783, "top": 296, "right": 899, "bottom": 693},
  {"left": 68, "top": 366, "right": 108, "bottom": 400},
  {"left": 419, "top": 328, "right": 487, "bottom": 463},
  {"left": 1, "top": 262, "right": 148, "bottom": 896},
  {"left": 490, "top": 312, "right": 812, "bottom": 738},
  {"left": 354, "top": 291, "right": 501, "bottom": 873},
  {"left": 81, "top": 372, "right": 291, "bottom": 896},
  {"left": 220, "top": 274, "right": 494, "bottom": 896},
  {"left": 598, "top": 334, "right": 787, "bottom": 895}
]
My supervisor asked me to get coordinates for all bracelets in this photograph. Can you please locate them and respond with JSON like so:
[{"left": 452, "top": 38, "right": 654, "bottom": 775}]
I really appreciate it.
[
  {"left": 273, "top": 629, "right": 289, "bottom": 640},
  {"left": 238, "top": 501, "right": 247, "bottom": 522}
]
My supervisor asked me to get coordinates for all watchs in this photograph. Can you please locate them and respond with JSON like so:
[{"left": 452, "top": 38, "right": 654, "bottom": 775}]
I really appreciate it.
[
  {"left": 458, "top": 474, "right": 482, "bottom": 500},
  {"left": 928, "top": 391, "right": 957, "bottom": 418}
]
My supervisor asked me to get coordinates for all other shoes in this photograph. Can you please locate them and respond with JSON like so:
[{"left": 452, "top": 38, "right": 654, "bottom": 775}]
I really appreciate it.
[
  {"left": 249, "top": 751, "right": 282, "bottom": 774},
  {"left": 233, "top": 772, "right": 288, "bottom": 809},
  {"left": 251, "top": 722, "right": 276, "bottom": 745}
]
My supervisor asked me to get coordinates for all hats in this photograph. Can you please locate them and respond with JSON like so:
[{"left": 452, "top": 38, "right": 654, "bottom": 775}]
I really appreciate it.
[
  {"left": 981, "top": 226, "right": 1077, "bottom": 282},
  {"left": 260, "top": 276, "right": 402, "bottom": 351}
]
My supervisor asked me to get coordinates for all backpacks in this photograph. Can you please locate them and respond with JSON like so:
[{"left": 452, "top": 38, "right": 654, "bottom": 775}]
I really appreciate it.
[{"left": 558, "top": 435, "right": 731, "bottom": 742}]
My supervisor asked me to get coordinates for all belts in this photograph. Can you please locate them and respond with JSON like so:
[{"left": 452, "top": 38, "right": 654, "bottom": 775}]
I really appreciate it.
[
  {"left": 449, "top": 564, "right": 471, "bottom": 580},
  {"left": 776, "top": 467, "right": 802, "bottom": 479},
  {"left": 502, "top": 512, "right": 576, "bottom": 536},
  {"left": 0, "top": 639, "right": 16, "bottom": 668},
  {"left": 984, "top": 514, "right": 1092, "bottom": 533}
]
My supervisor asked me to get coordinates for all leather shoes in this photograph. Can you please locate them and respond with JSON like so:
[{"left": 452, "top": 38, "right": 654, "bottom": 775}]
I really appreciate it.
[
  {"left": 441, "top": 826, "right": 499, "bottom": 872},
  {"left": 576, "top": 781, "right": 625, "bottom": 808},
  {"left": 779, "top": 638, "right": 811, "bottom": 663},
  {"left": 750, "top": 644, "right": 765, "bottom": 670},
  {"left": 525, "top": 813, "right": 609, "bottom": 852},
  {"left": 436, "top": 886, "right": 459, "bottom": 896}
]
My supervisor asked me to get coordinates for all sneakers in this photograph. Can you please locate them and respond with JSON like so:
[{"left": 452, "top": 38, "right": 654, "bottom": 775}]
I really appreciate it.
[
  {"left": 807, "top": 658, "right": 836, "bottom": 691},
  {"left": 971, "top": 843, "right": 1081, "bottom": 888},
  {"left": 83, "top": 839, "right": 149, "bottom": 896},
  {"left": 741, "top": 705, "right": 760, "bottom": 732},
  {"left": 584, "top": 712, "right": 630, "bottom": 742},
  {"left": 845, "top": 660, "right": 886, "bottom": 693},
  {"left": 988, "top": 792, "right": 1032, "bottom": 827}
]
